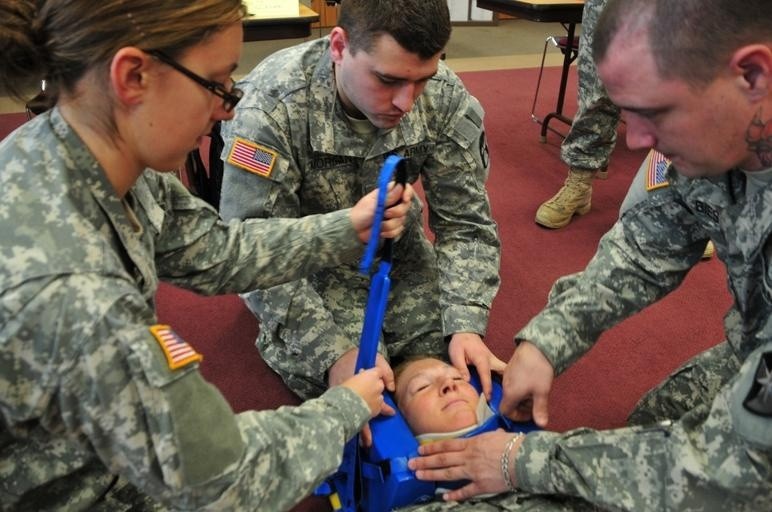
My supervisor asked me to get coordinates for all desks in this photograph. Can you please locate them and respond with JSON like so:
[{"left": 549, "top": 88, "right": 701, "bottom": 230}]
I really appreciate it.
[
  {"left": 183, "top": 0, "right": 323, "bottom": 223},
  {"left": 475, "top": 0, "right": 610, "bottom": 180}
]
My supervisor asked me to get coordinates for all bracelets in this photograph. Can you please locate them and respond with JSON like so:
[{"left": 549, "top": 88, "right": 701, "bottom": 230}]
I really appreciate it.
[{"left": 500, "top": 430, "right": 524, "bottom": 496}]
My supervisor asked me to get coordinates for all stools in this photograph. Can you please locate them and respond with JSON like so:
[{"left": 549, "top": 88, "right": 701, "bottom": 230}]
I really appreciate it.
[
  {"left": 25, "top": 89, "right": 54, "bottom": 120},
  {"left": 529, "top": 35, "right": 580, "bottom": 138}
]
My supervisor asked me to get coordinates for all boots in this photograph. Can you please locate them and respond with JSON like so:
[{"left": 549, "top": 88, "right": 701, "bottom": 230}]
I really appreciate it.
[{"left": 535, "top": 169, "right": 596, "bottom": 230}]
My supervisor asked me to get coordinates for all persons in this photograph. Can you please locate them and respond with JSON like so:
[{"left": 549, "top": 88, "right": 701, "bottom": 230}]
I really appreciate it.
[
  {"left": 220, "top": 0, "right": 511, "bottom": 452},
  {"left": 533, "top": 0, "right": 717, "bottom": 262},
  {"left": 0, "top": 0, "right": 414, "bottom": 511},
  {"left": 405, "top": 0, "right": 771, "bottom": 511},
  {"left": 384, "top": 350, "right": 599, "bottom": 512}
]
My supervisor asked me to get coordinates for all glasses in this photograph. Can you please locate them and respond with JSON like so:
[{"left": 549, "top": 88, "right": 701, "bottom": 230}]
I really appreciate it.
[{"left": 143, "top": 48, "right": 244, "bottom": 112}]
